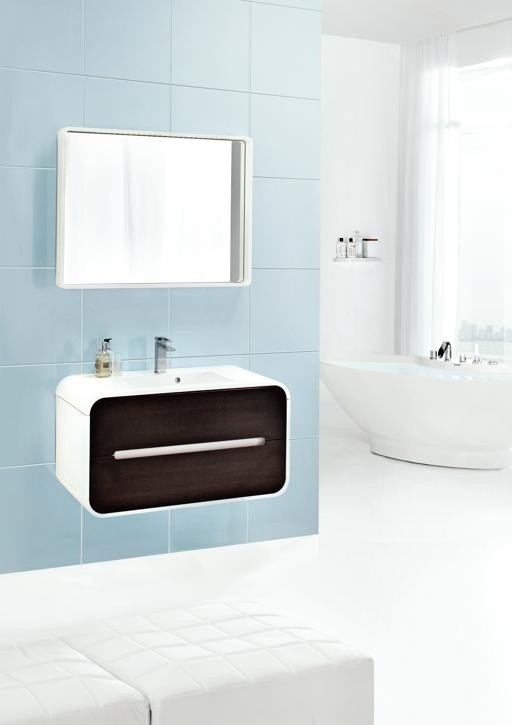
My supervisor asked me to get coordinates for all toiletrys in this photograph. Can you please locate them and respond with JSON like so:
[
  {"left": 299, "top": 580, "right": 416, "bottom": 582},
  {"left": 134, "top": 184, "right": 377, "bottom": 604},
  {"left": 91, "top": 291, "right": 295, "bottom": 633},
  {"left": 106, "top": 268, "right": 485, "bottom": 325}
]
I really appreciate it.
[
  {"left": 95, "top": 338, "right": 113, "bottom": 377},
  {"left": 338, "top": 238, "right": 357, "bottom": 258}
]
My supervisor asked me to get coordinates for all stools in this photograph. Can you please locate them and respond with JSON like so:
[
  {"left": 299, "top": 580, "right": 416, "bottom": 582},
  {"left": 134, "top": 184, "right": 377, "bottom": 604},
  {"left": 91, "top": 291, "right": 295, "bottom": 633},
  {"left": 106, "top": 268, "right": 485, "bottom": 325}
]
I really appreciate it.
[
  {"left": 0, "top": 626, "right": 151, "bottom": 725},
  {"left": 40, "top": 600, "right": 375, "bottom": 725}
]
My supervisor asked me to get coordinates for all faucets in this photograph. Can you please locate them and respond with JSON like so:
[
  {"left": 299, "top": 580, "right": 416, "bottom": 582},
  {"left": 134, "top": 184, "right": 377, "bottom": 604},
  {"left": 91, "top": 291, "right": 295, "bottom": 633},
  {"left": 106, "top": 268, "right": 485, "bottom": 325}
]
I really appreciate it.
[
  {"left": 437, "top": 341, "right": 452, "bottom": 364},
  {"left": 154, "top": 337, "right": 176, "bottom": 373}
]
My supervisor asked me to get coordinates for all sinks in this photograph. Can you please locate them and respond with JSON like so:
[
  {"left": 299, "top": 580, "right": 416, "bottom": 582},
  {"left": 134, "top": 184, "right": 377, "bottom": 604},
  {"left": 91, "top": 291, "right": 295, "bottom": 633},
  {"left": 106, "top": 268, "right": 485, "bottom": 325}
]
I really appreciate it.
[{"left": 118, "top": 372, "right": 234, "bottom": 391}]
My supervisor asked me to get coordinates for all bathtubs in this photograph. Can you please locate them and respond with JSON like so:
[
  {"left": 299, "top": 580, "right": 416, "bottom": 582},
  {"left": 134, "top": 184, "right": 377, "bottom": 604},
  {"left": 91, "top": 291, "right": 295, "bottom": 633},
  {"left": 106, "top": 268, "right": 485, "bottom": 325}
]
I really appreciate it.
[{"left": 320, "top": 354, "right": 512, "bottom": 468}]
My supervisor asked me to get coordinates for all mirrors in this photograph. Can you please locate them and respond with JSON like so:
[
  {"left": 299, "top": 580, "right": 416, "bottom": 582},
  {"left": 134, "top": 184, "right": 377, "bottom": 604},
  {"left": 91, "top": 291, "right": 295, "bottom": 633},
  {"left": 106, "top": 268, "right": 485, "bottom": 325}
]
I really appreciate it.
[{"left": 54, "top": 126, "right": 254, "bottom": 291}]
